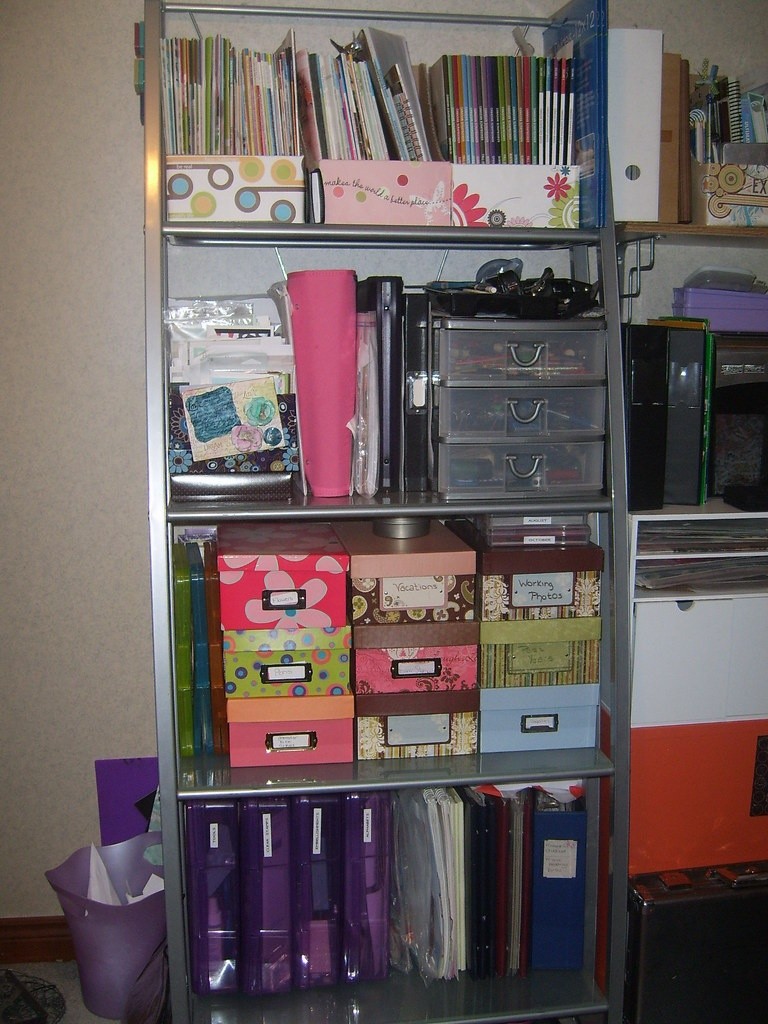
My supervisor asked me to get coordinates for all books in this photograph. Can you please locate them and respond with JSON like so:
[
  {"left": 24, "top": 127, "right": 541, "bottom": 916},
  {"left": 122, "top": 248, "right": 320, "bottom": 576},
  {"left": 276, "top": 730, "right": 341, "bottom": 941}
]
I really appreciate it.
[
  {"left": 392, "top": 787, "right": 533, "bottom": 981},
  {"left": 160, "top": 0, "right": 768, "bottom": 229}
]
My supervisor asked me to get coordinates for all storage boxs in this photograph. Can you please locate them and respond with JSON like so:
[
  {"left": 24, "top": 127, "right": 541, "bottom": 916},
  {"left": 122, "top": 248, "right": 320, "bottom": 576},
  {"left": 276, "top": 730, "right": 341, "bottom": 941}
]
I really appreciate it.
[
  {"left": 607, "top": 28, "right": 692, "bottom": 223},
  {"left": 673, "top": 287, "right": 768, "bottom": 335},
  {"left": 215, "top": 523, "right": 605, "bottom": 768},
  {"left": 629, "top": 600, "right": 768, "bottom": 878},
  {"left": 167, "top": 154, "right": 581, "bottom": 230},
  {"left": 171, "top": 393, "right": 301, "bottom": 501},
  {"left": 688, "top": 73, "right": 768, "bottom": 229},
  {"left": 185, "top": 793, "right": 397, "bottom": 997}
]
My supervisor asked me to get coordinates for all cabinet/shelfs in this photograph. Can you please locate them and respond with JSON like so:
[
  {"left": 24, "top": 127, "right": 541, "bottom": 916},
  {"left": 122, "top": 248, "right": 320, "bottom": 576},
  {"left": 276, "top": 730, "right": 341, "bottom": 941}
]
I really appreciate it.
[
  {"left": 143, "top": 0, "right": 629, "bottom": 1024},
  {"left": 615, "top": 222, "right": 768, "bottom": 1024},
  {"left": 430, "top": 318, "right": 608, "bottom": 501}
]
